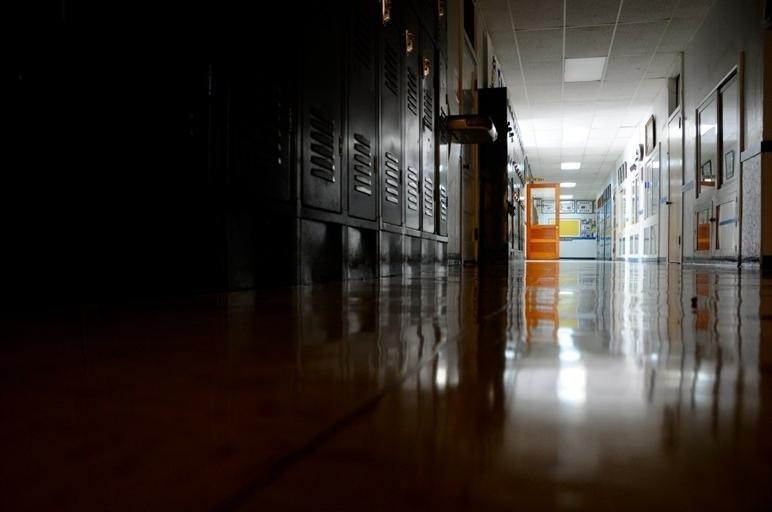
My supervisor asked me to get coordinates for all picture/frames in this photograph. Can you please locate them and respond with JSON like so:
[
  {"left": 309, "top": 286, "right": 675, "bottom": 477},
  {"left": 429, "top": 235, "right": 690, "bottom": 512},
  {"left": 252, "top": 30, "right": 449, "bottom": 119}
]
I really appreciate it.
[
  {"left": 644, "top": 114, "right": 656, "bottom": 156},
  {"left": 540, "top": 198, "right": 595, "bottom": 215},
  {"left": 700, "top": 150, "right": 735, "bottom": 181}
]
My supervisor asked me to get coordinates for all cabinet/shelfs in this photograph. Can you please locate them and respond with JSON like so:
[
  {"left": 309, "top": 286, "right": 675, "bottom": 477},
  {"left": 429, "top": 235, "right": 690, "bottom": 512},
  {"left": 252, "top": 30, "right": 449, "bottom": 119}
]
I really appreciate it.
[
  {"left": 292, "top": 0, "right": 457, "bottom": 286},
  {"left": 477, "top": 84, "right": 531, "bottom": 264}
]
